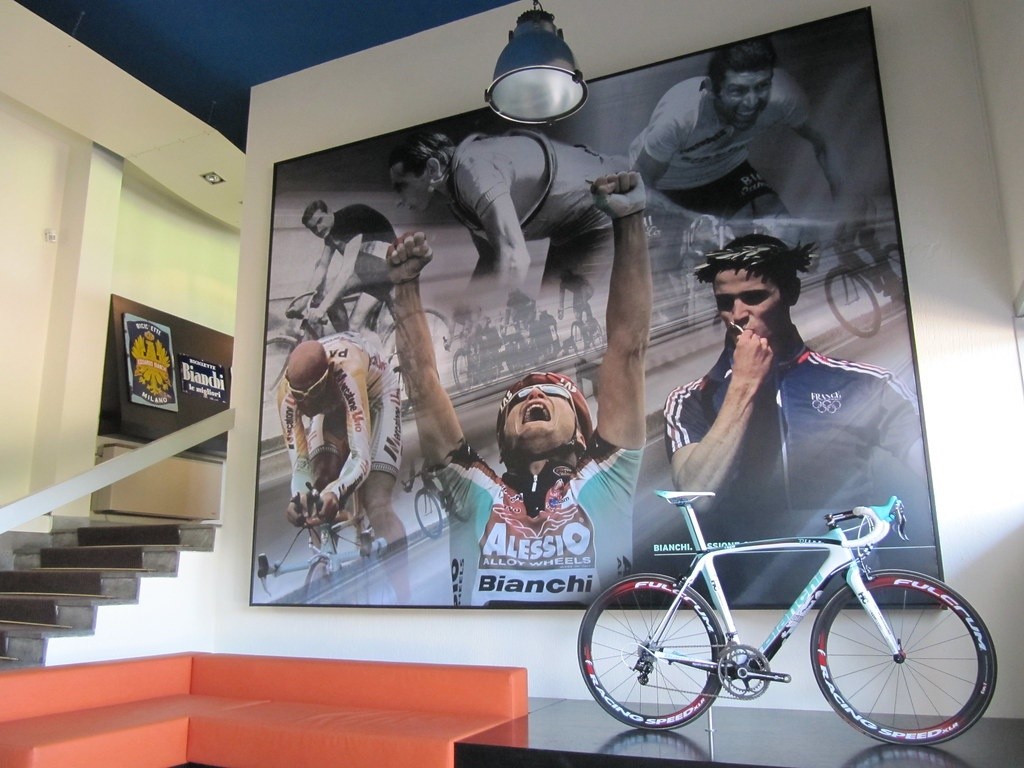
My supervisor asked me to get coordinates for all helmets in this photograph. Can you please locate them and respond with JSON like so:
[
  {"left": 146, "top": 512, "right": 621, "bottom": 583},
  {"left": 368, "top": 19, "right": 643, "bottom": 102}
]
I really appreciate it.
[
  {"left": 497, "top": 372, "right": 592, "bottom": 447},
  {"left": 287, "top": 340, "right": 329, "bottom": 396}
]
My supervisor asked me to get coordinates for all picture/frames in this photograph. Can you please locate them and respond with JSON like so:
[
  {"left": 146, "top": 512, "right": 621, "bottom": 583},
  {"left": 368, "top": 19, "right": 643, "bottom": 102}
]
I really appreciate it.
[{"left": 249, "top": 6, "right": 947, "bottom": 612}]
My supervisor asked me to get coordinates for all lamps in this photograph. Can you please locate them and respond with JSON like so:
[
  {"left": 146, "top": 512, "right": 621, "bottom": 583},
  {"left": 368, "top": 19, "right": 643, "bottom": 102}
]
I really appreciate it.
[{"left": 484, "top": 0, "right": 590, "bottom": 127}]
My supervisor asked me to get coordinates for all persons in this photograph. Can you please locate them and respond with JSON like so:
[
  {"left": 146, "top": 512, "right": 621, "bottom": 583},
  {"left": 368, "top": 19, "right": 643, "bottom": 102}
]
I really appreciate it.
[
  {"left": 554, "top": 266, "right": 595, "bottom": 335},
  {"left": 664, "top": 232, "right": 937, "bottom": 608},
  {"left": 441, "top": 301, "right": 492, "bottom": 361},
  {"left": 612, "top": 35, "right": 856, "bottom": 304},
  {"left": 500, "top": 286, "right": 540, "bottom": 343},
  {"left": 295, "top": 199, "right": 403, "bottom": 345},
  {"left": 379, "top": 128, "right": 656, "bottom": 340},
  {"left": 384, "top": 168, "right": 652, "bottom": 608},
  {"left": 274, "top": 327, "right": 413, "bottom": 606}
]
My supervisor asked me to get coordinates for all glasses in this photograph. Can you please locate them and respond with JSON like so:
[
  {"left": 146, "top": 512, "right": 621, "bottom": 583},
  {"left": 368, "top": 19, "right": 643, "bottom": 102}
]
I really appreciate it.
[
  {"left": 715, "top": 287, "right": 775, "bottom": 311},
  {"left": 507, "top": 384, "right": 577, "bottom": 420},
  {"left": 288, "top": 368, "right": 329, "bottom": 402}
]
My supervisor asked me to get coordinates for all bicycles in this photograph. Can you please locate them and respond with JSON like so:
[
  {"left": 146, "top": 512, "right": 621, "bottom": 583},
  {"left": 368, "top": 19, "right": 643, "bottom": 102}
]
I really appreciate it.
[
  {"left": 265, "top": 287, "right": 455, "bottom": 419},
  {"left": 256, "top": 477, "right": 407, "bottom": 608},
  {"left": 404, "top": 459, "right": 478, "bottom": 540},
  {"left": 819, "top": 225, "right": 912, "bottom": 340},
  {"left": 437, "top": 300, "right": 612, "bottom": 392},
  {"left": 577, "top": 486, "right": 1001, "bottom": 762}
]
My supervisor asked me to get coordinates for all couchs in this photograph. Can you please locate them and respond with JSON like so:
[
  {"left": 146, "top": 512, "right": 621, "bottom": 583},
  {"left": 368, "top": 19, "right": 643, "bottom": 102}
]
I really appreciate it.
[{"left": 0, "top": 650, "right": 529, "bottom": 768}]
